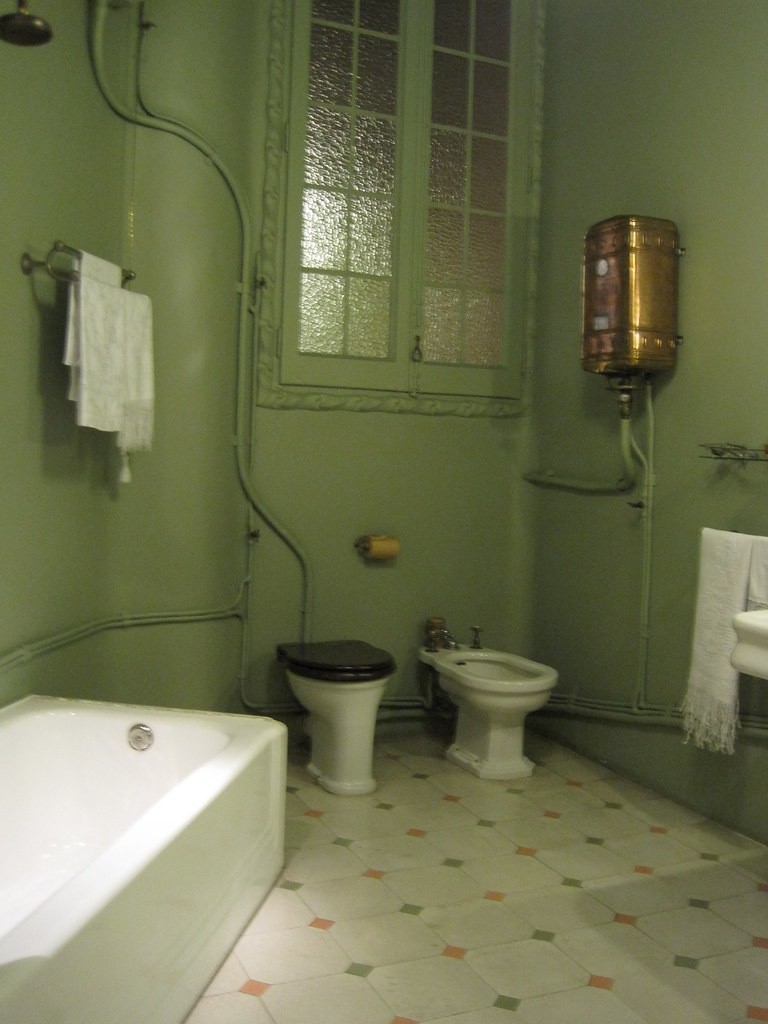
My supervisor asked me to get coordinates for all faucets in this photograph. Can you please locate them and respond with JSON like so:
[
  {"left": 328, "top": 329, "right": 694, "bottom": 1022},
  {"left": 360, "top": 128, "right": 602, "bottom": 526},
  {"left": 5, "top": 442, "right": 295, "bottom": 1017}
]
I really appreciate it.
[{"left": 424, "top": 624, "right": 459, "bottom": 654}]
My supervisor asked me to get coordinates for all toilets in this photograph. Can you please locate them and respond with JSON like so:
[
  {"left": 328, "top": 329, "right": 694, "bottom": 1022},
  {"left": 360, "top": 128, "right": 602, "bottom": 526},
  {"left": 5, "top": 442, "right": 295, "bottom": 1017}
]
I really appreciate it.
[{"left": 275, "top": 637, "right": 399, "bottom": 796}]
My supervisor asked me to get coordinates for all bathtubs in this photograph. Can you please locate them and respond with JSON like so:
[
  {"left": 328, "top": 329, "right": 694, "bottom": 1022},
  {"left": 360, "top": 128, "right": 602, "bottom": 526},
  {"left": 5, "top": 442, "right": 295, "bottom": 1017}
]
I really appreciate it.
[{"left": 0, "top": 694, "right": 286, "bottom": 1023}]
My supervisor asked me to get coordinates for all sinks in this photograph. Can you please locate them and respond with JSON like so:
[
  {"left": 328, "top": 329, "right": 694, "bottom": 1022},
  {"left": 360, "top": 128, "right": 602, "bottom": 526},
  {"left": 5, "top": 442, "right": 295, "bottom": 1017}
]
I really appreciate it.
[
  {"left": 731, "top": 610, "right": 767, "bottom": 684},
  {"left": 416, "top": 617, "right": 560, "bottom": 780}
]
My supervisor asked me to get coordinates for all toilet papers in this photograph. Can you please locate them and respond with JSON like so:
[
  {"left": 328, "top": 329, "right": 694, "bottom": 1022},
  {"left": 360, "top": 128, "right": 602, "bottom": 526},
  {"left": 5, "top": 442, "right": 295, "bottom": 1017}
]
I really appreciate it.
[{"left": 359, "top": 534, "right": 400, "bottom": 561}]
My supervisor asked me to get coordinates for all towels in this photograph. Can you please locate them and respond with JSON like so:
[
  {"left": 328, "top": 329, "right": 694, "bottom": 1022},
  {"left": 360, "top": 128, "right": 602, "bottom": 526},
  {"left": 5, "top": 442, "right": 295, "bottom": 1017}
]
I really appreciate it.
[
  {"left": 677, "top": 526, "right": 768, "bottom": 754},
  {"left": 61, "top": 248, "right": 154, "bottom": 453}
]
